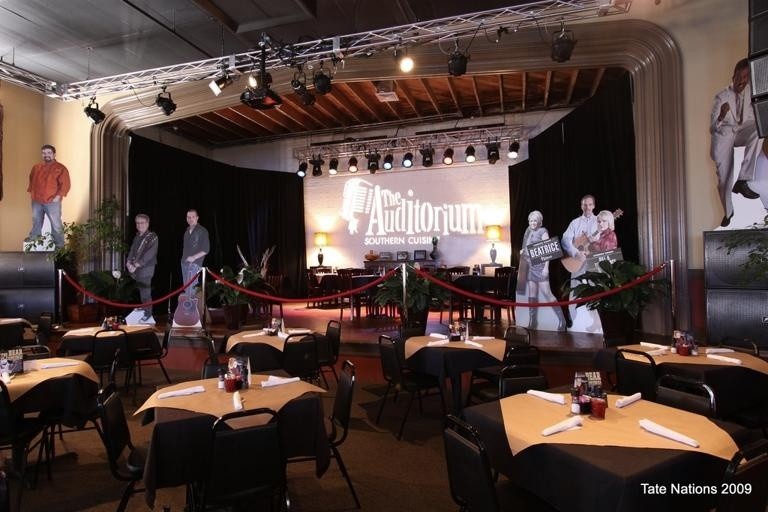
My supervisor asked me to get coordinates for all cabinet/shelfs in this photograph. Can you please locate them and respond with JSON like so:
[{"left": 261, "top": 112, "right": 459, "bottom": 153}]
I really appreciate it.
[
  {"left": 703, "top": 230, "right": 768, "bottom": 355},
  {"left": 0, "top": 250, "right": 55, "bottom": 317}
]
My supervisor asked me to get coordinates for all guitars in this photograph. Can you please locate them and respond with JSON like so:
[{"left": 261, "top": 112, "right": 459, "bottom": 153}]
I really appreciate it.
[
  {"left": 561, "top": 208, "right": 623, "bottom": 273},
  {"left": 121, "top": 232, "right": 158, "bottom": 281},
  {"left": 173, "top": 262, "right": 200, "bottom": 326},
  {"left": 517, "top": 229, "right": 531, "bottom": 295}
]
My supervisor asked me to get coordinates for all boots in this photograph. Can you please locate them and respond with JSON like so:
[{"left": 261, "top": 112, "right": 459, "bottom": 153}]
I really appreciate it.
[
  {"left": 526, "top": 298, "right": 538, "bottom": 330},
  {"left": 551, "top": 300, "right": 566, "bottom": 331}
]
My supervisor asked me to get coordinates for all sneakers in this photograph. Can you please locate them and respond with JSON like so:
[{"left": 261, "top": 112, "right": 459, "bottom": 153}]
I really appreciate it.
[
  {"left": 138, "top": 315, "right": 149, "bottom": 324},
  {"left": 135, "top": 308, "right": 143, "bottom": 311}
]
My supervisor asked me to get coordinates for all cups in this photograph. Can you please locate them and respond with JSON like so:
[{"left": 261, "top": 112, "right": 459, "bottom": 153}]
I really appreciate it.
[
  {"left": 112, "top": 322, "right": 119, "bottom": 331},
  {"left": 224, "top": 373, "right": 238, "bottom": 393},
  {"left": 679, "top": 343, "right": 689, "bottom": 356},
  {"left": 590, "top": 397, "right": 607, "bottom": 421}
]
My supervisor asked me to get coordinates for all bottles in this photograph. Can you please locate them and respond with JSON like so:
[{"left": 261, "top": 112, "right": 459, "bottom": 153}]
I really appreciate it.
[
  {"left": 108, "top": 321, "right": 113, "bottom": 331},
  {"left": 691, "top": 344, "right": 698, "bottom": 356},
  {"left": 459, "top": 324, "right": 466, "bottom": 340},
  {"left": 217, "top": 374, "right": 224, "bottom": 389},
  {"left": 570, "top": 396, "right": 581, "bottom": 415},
  {"left": 670, "top": 342, "right": 678, "bottom": 354},
  {"left": 447, "top": 325, "right": 454, "bottom": 338}
]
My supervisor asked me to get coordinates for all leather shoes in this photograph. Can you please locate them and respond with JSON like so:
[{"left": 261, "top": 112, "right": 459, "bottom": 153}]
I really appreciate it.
[
  {"left": 732, "top": 181, "right": 760, "bottom": 199},
  {"left": 721, "top": 210, "right": 733, "bottom": 226}
]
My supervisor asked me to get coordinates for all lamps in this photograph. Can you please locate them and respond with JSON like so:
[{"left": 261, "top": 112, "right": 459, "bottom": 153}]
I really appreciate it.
[
  {"left": 296, "top": 141, "right": 519, "bottom": 177},
  {"left": 485, "top": 225, "right": 501, "bottom": 264},
  {"left": 313, "top": 232, "right": 326, "bottom": 268}
]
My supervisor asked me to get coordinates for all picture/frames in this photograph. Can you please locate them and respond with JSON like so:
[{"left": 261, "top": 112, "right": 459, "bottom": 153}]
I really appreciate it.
[
  {"left": 414, "top": 250, "right": 426, "bottom": 261},
  {"left": 396, "top": 251, "right": 408, "bottom": 261}
]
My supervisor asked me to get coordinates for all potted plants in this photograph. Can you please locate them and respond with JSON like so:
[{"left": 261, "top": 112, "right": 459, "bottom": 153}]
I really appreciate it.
[
  {"left": 374, "top": 261, "right": 450, "bottom": 334},
  {"left": 24, "top": 193, "right": 129, "bottom": 321},
  {"left": 79, "top": 271, "right": 154, "bottom": 317},
  {"left": 561, "top": 260, "right": 670, "bottom": 347},
  {"left": 205, "top": 267, "right": 264, "bottom": 329}
]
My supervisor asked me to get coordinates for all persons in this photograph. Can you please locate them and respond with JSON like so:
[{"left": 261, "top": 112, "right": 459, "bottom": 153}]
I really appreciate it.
[
  {"left": 127, "top": 214, "right": 158, "bottom": 324},
  {"left": 566, "top": 210, "right": 618, "bottom": 334},
  {"left": 709, "top": 59, "right": 764, "bottom": 225},
  {"left": 520, "top": 211, "right": 565, "bottom": 330},
  {"left": 25, "top": 145, "right": 70, "bottom": 253},
  {"left": 560, "top": 194, "right": 603, "bottom": 322},
  {"left": 180, "top": 210, "right": 209, "bottom": 293}
]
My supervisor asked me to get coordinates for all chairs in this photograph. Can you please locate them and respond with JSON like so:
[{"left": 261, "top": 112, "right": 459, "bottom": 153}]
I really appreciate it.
[
  {"left": 305, "top": 260, "right": 395, "bottom": 320},
  {"left": 248, "top": 280, "right": 283, "bottom": 325},
  {"left": 437, "top": 266, "right": 519, "bottom": 324}
]
